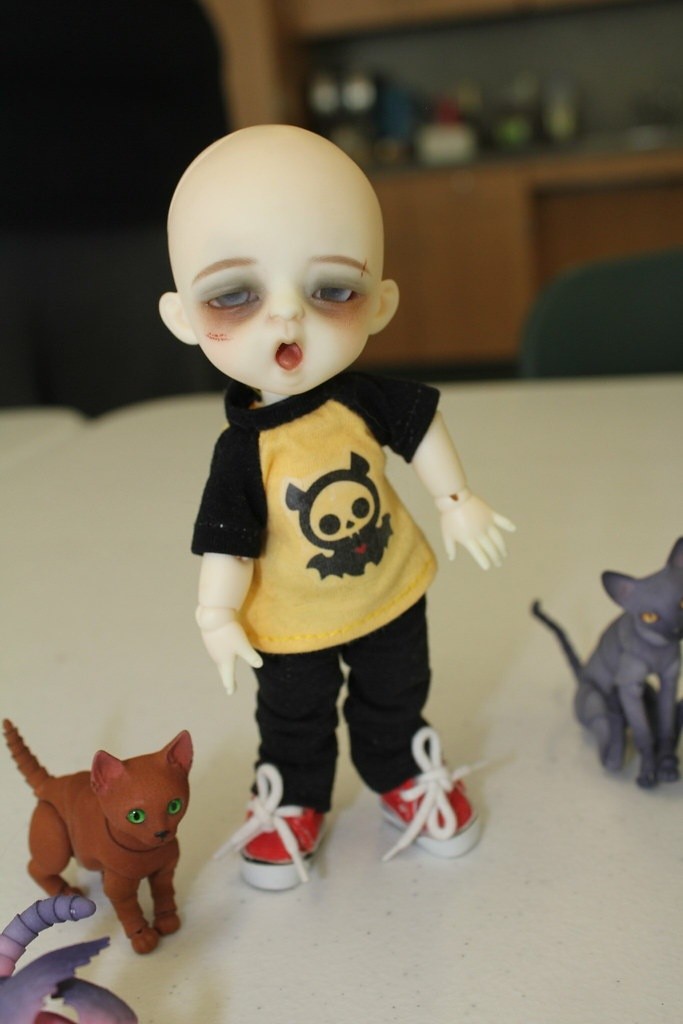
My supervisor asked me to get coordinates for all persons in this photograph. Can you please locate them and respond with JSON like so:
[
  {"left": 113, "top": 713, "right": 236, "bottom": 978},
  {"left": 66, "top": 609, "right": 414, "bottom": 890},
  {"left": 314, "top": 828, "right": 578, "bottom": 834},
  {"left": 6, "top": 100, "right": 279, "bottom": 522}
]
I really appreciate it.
[{"left": 156, "top": 125, "right": 517, "bottom": 893}]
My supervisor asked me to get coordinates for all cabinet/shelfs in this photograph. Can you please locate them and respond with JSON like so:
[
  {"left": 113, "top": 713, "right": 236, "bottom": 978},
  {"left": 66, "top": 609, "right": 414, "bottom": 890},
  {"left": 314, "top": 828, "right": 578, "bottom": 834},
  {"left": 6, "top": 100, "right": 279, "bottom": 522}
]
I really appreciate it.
[{"left": 206, "top": 1, "right": 682, "bottom": 366}]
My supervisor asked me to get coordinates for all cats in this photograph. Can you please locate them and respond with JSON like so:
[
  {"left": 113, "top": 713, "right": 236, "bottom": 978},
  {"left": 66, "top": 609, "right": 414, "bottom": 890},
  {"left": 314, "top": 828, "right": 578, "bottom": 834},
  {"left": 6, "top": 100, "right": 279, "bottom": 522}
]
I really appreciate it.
[
  {"left": 522, "top": 534, "right": 683, "bottom": 788},
  {"left": 1, "top": 719, "right": 194, "bottom": 953}
]
